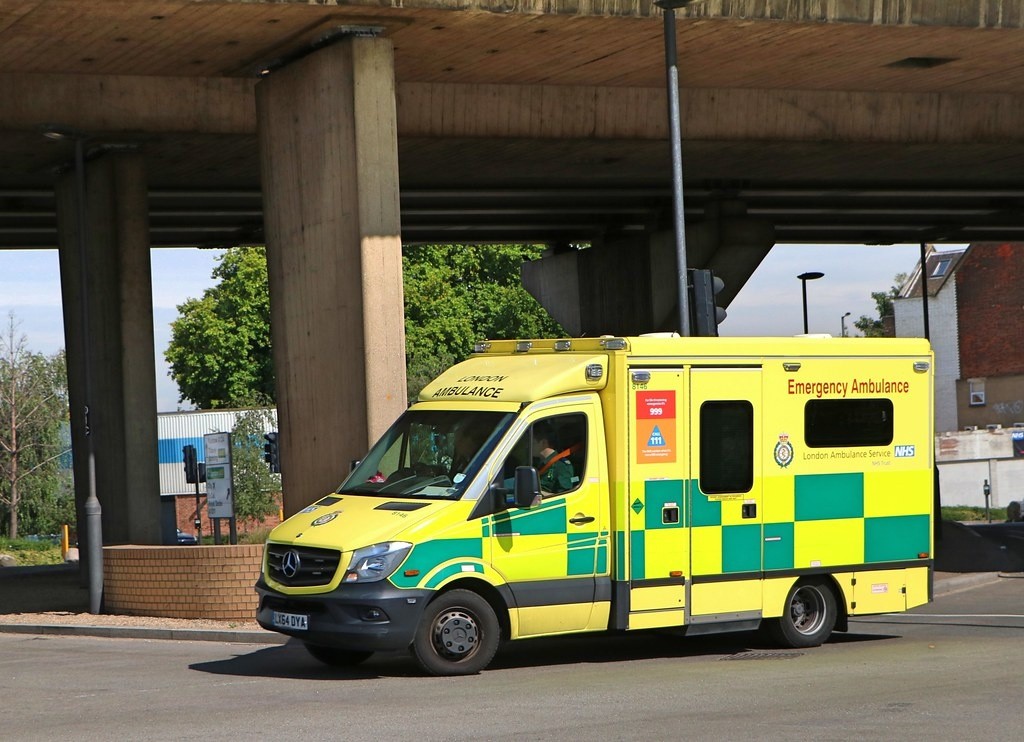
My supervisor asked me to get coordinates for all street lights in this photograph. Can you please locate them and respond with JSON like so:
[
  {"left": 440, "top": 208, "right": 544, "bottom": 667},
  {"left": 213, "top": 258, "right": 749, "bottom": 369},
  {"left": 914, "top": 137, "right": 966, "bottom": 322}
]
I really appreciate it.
[{"left": 799, "top": 272, "right": 827, "bottom": 334}]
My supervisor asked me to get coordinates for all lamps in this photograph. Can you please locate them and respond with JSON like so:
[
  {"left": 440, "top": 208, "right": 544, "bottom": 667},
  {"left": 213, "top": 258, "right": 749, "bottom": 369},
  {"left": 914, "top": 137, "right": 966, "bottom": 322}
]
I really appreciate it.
[{"left": 983, "top": 484, "right": 990, "bottom": 494}]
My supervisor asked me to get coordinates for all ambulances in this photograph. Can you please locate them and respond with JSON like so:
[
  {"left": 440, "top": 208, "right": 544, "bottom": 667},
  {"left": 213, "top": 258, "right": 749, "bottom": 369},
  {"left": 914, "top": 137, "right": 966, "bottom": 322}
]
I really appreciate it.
[{"left": 252, "top": 328, "right": 939, "bottom": 679}]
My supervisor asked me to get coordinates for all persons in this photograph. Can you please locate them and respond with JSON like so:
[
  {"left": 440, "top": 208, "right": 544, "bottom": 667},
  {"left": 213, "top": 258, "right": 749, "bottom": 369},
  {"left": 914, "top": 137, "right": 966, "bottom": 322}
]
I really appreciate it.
[
  {"left": 447, "top": 424, "right": 505, "bottom": 488},
  {"left": 505, "top": 427, "right": 573, "bottom": 503},
  {"left": 1006, "top": 502, "right": 1023, "bottom": 522}
]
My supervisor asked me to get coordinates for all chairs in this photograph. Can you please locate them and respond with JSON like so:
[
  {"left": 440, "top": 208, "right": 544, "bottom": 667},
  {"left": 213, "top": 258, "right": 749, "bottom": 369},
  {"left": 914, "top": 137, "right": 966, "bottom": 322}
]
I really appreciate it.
[{"left": 548, "top": 424, "right": 584, "bottom": 486}]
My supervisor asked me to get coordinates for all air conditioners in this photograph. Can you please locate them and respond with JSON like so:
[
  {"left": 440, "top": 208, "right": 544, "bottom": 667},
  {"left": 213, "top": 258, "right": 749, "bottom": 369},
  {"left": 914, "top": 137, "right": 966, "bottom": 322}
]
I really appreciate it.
[
  {"left": 1014, "top": 423, "right": 1024, "bottom": 427},
  {"left": 964, "top": 426, "right": 978, "bottom": 431},
  {"left": 986, "top": 424, "right": 1001, "bottom": 429}
]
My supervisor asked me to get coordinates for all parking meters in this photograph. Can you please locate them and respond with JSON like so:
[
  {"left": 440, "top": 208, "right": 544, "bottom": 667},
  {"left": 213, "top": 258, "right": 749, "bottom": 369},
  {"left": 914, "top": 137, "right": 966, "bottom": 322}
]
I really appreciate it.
[{"left": 983, "top": 479, "right": 991, "bottom": 522}]
